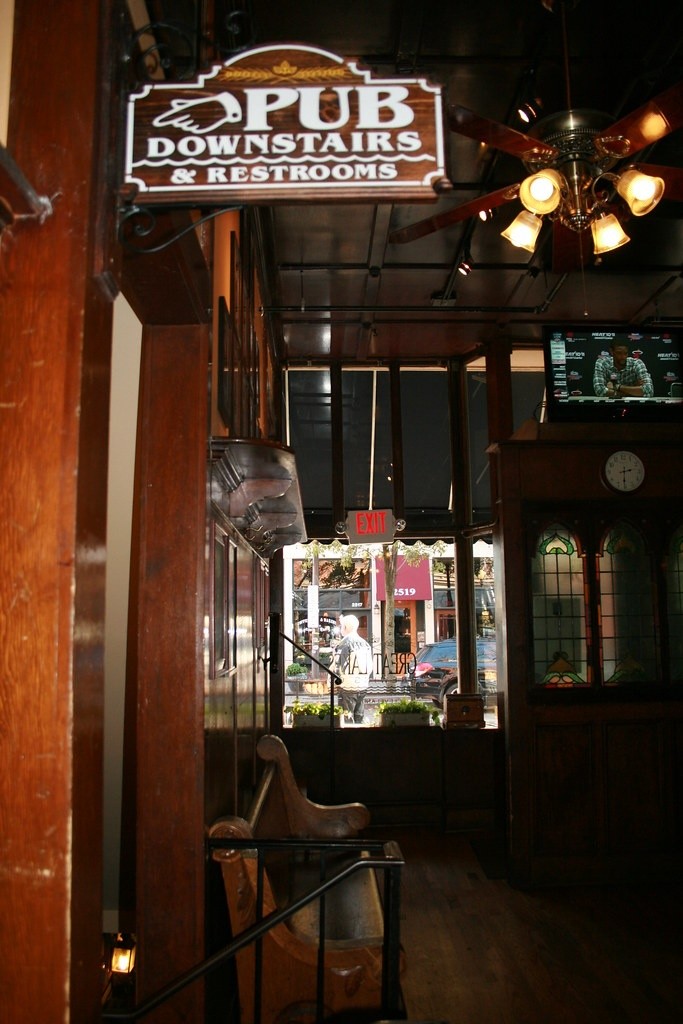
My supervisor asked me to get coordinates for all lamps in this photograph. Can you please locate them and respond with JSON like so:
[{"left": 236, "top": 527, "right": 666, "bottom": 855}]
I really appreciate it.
[{"left": 503, "top": 136, "right": 668, "bottom": 256}]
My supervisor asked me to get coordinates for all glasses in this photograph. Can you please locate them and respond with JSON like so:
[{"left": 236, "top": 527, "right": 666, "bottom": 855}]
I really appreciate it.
[{"left": 614, "top": 350, "right": 631, "bottom": 356}]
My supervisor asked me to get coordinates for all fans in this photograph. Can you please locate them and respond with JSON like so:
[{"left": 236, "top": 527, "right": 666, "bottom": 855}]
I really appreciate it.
[{"left": 386, "top": 0, "right": 683, "bottom": 275}]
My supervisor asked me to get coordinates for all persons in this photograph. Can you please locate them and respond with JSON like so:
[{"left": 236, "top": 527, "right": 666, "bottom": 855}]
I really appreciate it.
[
  {"left": 593, "top": 343, "right": 654, "bottom": 397},
  {"left": 329, "top": 614, "right": 372, "bottom": 723}
]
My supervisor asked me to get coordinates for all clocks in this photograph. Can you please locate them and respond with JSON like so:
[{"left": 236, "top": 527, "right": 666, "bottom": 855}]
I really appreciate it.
[{"left": 599, "top": 446, "right": 648, "bottom": 495}]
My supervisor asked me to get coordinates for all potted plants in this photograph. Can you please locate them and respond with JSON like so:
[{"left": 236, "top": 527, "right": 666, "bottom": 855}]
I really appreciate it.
[
  {"left": 378, "top": 699, "right": 441, "bottom": 727},
  {"left": 286, "top": 663, "right": 308, "bottom": 679},
  {"left": 284, "top": 701, "right": 345, "bottom": 729}
]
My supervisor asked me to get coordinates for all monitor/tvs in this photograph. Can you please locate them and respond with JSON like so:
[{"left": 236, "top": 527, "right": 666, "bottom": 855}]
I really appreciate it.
[{"left": 541, "top": 323, "right": 683, "bottom": 425}]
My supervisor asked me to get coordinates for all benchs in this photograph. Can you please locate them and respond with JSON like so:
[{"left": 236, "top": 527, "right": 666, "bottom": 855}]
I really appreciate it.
[{"left": 208, "top": 734, "right": 406, "bottom": 1024}]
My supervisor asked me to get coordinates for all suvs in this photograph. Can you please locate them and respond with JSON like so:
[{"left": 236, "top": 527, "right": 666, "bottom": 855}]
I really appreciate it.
[{"left": 399, "top": 639, "right": 497, "bottom": 711}]
